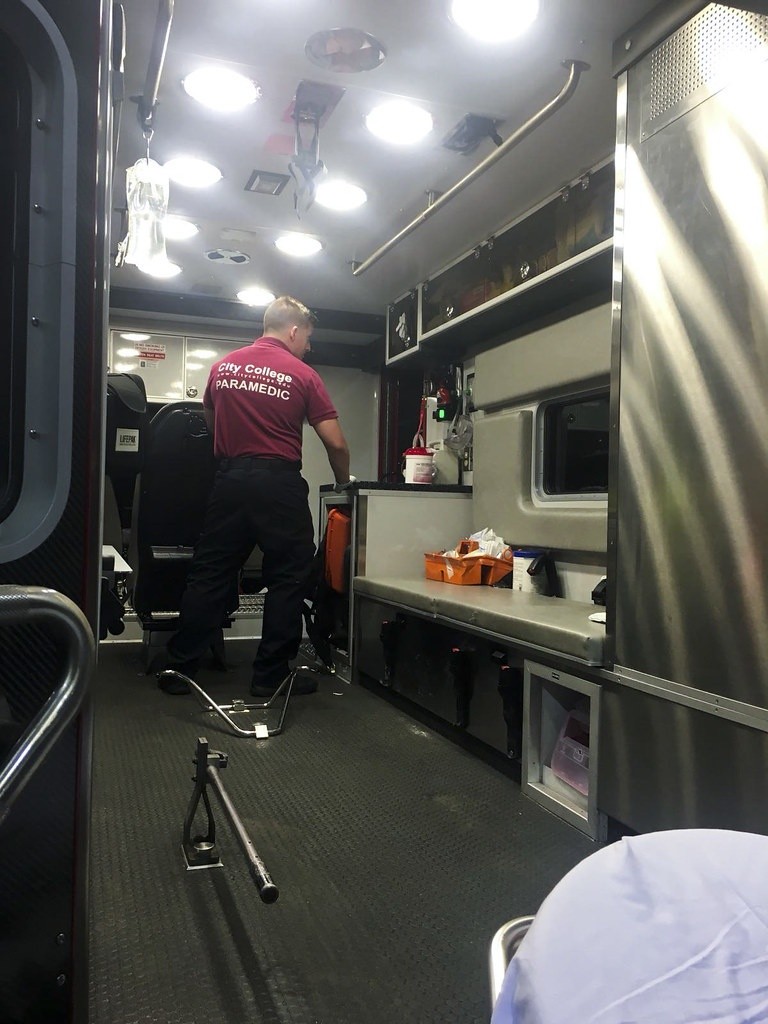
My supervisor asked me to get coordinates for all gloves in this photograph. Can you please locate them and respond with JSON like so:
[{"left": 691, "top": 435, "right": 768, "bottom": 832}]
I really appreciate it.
[{"left": 334, "top": 480, "right": 352, "bottom": 494}]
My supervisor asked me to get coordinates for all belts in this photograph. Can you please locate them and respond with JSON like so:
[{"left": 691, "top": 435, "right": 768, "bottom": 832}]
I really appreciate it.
[{"left": 228, "top": 458, "right": 296, "bottom": 471}]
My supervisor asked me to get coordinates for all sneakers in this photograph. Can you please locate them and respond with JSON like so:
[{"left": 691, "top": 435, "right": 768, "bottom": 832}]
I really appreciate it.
[
  {"left": 250, "top": 669, "right": 319, "bottom": 697},
  {"left": 157, "top": 670, "right": 191, "bottom": 695}
]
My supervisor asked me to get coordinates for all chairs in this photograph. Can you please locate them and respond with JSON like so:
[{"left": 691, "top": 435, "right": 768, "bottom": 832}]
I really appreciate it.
[{"left": 129, "top": 400, "right": 240, "bottom": 670}]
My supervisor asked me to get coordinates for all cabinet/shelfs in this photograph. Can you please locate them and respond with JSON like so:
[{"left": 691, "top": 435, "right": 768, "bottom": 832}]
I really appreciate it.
[{"left": 317, "top": 480, "right": 473, "bottom": 689}]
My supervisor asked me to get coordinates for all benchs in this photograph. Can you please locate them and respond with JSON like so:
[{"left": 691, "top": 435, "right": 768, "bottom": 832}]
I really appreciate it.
[{"left": 351, "top": 565, "right": 606, "bottom": 786}]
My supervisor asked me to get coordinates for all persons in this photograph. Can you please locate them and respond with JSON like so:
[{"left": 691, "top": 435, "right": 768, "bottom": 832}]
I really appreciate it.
[{"left": 155, "top": 293, "right": 358, "bottom": 695}]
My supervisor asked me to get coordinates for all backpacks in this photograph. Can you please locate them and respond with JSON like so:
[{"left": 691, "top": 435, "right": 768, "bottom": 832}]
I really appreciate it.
[{"left": 106, "top": 374, "right": 147, "bottom": 452}]
